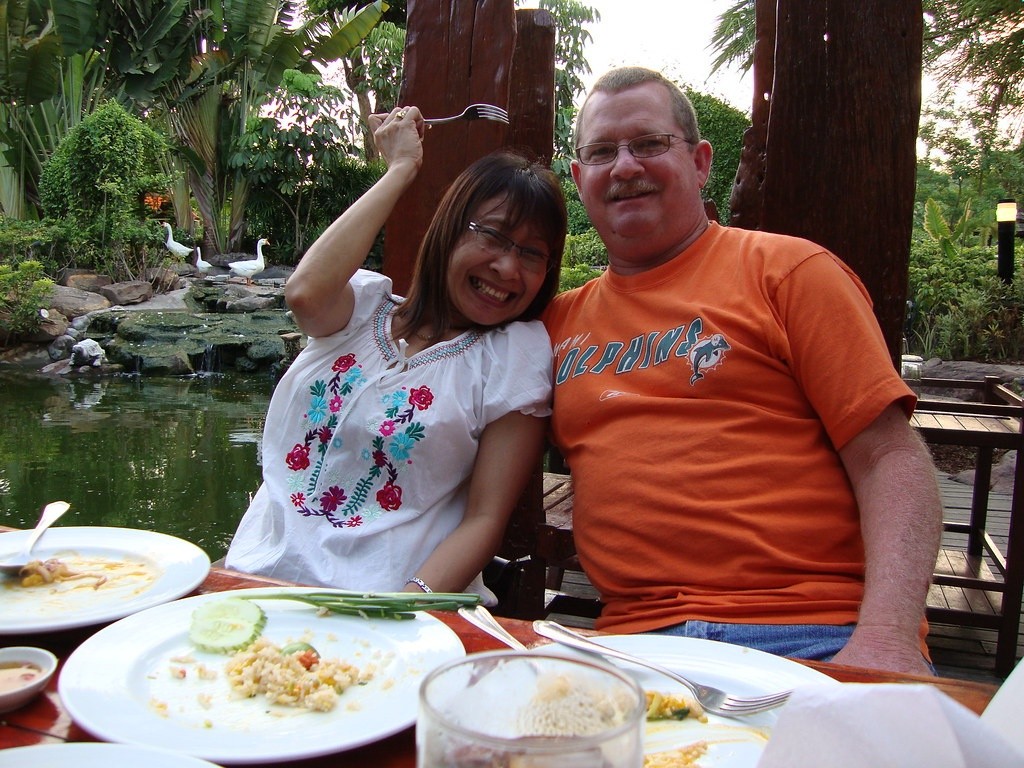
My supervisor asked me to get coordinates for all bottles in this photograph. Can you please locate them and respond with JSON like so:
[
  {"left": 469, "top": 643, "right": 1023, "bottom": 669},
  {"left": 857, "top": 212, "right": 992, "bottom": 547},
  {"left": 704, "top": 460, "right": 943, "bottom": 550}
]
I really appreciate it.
[{"left": 901, "top": 355, "right": 924, "bottom": 400}]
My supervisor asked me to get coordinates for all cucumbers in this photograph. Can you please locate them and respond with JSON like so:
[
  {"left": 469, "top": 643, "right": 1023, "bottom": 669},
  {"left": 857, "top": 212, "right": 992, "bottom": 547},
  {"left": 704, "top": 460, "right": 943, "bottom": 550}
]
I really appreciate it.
[{"left": 188, "top": 596, "right": 266, "bottom": 653}]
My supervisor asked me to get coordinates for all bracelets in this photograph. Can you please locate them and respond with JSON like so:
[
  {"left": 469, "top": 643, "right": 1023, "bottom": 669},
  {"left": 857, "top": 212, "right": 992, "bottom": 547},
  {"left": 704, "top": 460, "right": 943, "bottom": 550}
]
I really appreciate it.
[{"left": 405, "top": 577, "right": 432, "bottom": 593}]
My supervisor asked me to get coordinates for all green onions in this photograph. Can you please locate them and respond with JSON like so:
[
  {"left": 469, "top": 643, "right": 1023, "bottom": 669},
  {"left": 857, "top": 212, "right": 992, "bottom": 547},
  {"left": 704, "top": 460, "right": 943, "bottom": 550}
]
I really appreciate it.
[{"left": 229, "top": 589, "right": 481, "bottom": 620}]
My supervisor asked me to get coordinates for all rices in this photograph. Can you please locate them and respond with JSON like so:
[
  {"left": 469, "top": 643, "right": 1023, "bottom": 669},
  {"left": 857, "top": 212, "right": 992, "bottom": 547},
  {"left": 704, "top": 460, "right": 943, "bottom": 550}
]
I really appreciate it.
[{"left": 146, "top": 606, "right": 421, "bottom": 728}]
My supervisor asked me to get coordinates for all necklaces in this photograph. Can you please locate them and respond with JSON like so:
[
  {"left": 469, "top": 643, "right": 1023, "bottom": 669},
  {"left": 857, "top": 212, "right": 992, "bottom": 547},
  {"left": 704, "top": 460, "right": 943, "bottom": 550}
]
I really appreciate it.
[{"left": 416, "top": 333, "right": 432, "bottom": 340}]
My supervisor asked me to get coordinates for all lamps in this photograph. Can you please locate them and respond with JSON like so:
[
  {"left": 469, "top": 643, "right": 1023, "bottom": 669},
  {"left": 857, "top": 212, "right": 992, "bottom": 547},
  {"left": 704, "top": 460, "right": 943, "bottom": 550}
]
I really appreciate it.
[{"left": 995, "top": 197, "right": 1017, "bottom": 221}]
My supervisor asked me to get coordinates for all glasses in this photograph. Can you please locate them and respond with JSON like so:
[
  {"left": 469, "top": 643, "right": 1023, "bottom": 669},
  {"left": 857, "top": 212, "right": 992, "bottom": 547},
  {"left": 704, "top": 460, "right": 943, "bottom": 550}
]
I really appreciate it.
[
  {"left": 576, "top": 133, "right": 692, "bottom": 167},
  {"left": 463, "top": 218, "right": 555, "bottom": 274}
]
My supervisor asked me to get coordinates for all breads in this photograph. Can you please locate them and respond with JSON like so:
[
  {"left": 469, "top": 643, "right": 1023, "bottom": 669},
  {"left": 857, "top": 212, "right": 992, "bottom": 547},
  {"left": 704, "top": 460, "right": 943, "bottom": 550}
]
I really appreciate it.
[{"left": 515, "top": 681, "right": 643, "bottom": 766}]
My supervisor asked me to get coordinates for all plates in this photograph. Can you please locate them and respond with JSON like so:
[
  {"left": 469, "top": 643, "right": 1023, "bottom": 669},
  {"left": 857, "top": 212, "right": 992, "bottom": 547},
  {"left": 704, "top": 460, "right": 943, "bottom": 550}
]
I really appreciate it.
[
  {"left": 0, "top": 741, "right": 224, "bottom": 767},
  {"left": 57, "top": 586, "right": 466, "bottom": 766},
  {"left": 444, "top": 634, "right": 844, "bottom": 768},
  {"left": 0, "top": 525, "right": 211, "bottom": 635},
  {"left": 0, "top": 646, "right": 58, "bottom": 713}
]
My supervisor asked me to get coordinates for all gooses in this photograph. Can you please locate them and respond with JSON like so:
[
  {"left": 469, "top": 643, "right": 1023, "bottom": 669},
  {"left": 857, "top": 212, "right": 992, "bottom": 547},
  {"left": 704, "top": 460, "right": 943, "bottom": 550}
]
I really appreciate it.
[
  {"left": 160, "top": 221, "right": 194, "bottom": 261},
  {"left": 196, "top": 247, "right": 214, "bottom": 279},
  {"left": 228, "top": 238, "right": 271, "bottom": 286}
]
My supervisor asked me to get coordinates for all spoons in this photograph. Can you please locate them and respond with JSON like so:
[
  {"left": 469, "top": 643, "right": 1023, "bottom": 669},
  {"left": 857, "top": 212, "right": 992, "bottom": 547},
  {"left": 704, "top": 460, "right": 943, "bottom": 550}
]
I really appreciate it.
[{"left": 0, "top": 501, "right": 71, "bottom": 574}]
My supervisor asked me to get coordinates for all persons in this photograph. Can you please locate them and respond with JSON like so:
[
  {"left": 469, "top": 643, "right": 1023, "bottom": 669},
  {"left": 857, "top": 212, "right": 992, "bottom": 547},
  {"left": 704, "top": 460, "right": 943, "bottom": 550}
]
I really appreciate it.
[
  {"left": 544, "top": 66, "right": 942, "bottom": 675},
  {"left": 224, "top": 106, "right": 568, "bottom": 610}
]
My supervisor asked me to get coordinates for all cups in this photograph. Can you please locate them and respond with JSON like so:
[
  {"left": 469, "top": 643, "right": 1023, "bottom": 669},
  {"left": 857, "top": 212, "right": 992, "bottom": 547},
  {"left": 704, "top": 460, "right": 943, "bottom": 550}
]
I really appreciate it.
[{"left": 416, "top": 649, "right": 649, "bottom": 767}]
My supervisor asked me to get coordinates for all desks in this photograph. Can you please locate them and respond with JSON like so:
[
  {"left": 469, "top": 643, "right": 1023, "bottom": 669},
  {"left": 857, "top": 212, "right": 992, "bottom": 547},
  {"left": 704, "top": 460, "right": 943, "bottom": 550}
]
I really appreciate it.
[{"left": 0, "top": 567, "right": 1000, "bottom": 768}]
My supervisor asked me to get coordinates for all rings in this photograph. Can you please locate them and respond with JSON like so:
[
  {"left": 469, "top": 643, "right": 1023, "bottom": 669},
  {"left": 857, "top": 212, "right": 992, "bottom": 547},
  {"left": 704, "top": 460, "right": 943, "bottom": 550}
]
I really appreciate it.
[{"left": 396, "top": 110, "right": 407, "bottom": 119}]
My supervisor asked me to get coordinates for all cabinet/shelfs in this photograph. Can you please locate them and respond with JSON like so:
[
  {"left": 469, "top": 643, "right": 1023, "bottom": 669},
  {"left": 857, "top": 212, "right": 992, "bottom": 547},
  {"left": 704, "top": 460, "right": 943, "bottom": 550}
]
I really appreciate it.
[{"left": 543, "top": 374, "right": 1024, "bottom": 677}]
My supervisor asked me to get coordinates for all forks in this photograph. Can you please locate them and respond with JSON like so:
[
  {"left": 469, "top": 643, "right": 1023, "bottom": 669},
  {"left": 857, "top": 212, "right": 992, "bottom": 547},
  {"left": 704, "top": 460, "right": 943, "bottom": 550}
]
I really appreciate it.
[
  {"left": 424, "top": 103, "right": 510, "bottom": 125},
  {"left": 532, "top": 619, "right": 794, "bottom": 718}
]
